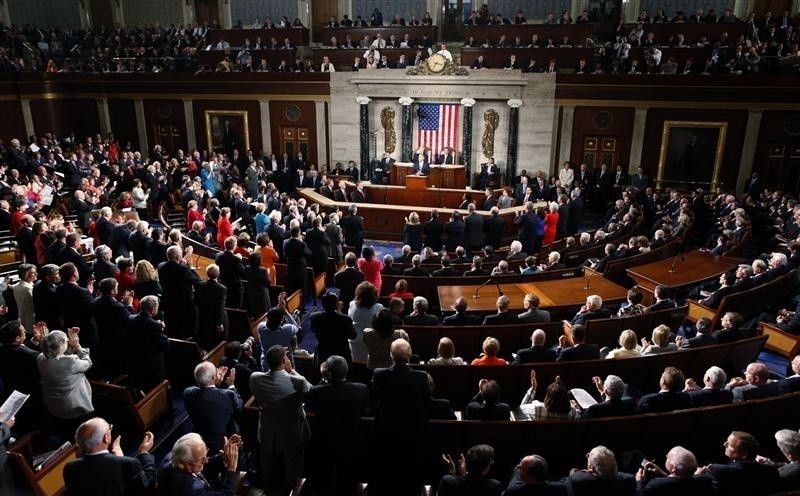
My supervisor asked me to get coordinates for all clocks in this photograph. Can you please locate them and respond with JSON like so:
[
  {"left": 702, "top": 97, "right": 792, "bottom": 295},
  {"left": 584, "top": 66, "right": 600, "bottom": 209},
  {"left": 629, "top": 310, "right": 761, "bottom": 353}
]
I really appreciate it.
[
  {"left": 784, "top": 114, "right": 800, "bottom": 135},
  {"left": 591, "top": 111, "right": 614, "bottom": 130},
  {"left": 285, "top": 104, "right": 302, "bottom": 121}
]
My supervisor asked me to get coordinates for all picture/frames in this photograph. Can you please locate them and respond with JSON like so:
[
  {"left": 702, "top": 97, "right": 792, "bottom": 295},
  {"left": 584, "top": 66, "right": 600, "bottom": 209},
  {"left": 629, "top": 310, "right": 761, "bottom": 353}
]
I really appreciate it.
[
  {"left": 204, "top": 109, "right": 250, "bottom": 157},
  {"left": 656, "top": 120, "right": 729, "bottom": 193}
]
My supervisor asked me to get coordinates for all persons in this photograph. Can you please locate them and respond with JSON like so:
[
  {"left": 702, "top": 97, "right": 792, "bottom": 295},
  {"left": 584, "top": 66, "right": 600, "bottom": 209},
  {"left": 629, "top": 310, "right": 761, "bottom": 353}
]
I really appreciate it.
[
  {"left": 1, "top": 1, "right": 800, "bottom": 77},
  {"left": 2, "top": 123, "right": 800, "bottom": 495}
]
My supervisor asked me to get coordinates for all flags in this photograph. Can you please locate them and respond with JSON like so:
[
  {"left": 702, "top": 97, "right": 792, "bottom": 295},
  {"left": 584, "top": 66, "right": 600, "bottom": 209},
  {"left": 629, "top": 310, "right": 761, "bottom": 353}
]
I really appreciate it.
[{"left": 414, "top": 100, "right": 466, "bottom": 164}]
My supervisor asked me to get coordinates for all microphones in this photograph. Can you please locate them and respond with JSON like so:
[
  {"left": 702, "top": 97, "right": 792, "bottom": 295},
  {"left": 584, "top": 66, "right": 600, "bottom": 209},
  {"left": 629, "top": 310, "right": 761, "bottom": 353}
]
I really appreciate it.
[
  {"left": 472, "top": 276, "right": 495, "bottom": 299},
  {"left": 583, "top": 264, "right": 601, "bottom": 290},
  {"left": 668, "top": 250, "right": 682, "bottom": 273},
  {"left": 194, "top": 249, "right": 200, "bottom": 270}
]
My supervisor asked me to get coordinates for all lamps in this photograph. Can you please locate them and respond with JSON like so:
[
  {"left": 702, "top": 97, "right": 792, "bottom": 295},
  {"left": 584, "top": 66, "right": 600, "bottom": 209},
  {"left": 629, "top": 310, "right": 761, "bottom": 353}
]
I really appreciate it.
[
  {"left": 398, "top": 96, "right": 415, "bottom": 106},
  {"left": 507, "top": 99, "right": 523, "bottom": 108},
  {"left": 460, "top": 97, "right": 476, "bottom": 107},
  {"left": 356, "top": 96, "right": 370, "bottom": 105}
]
